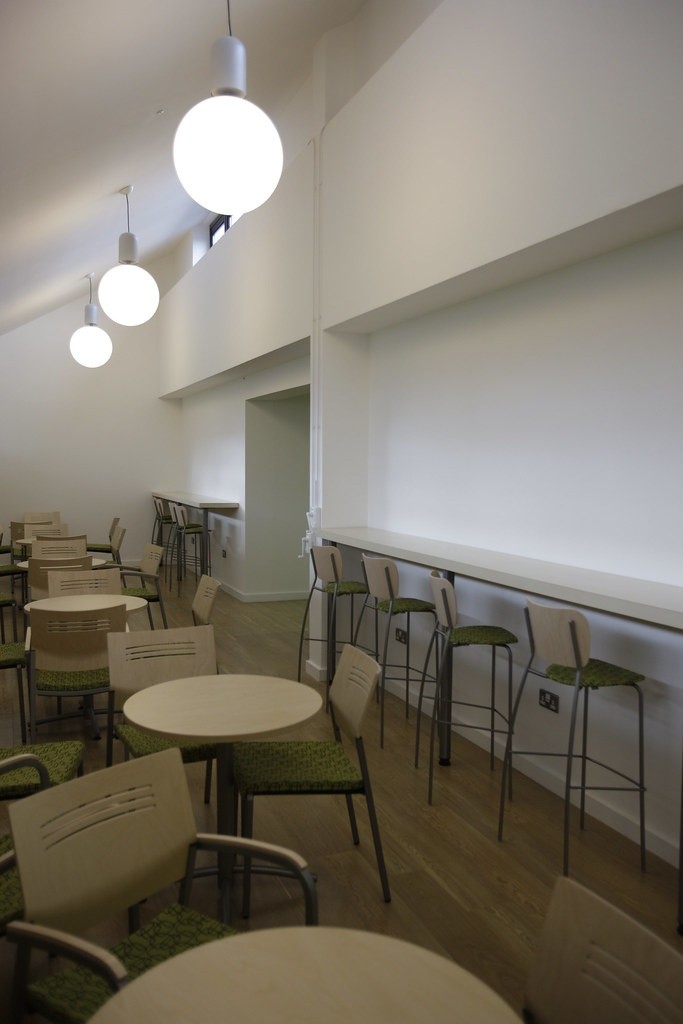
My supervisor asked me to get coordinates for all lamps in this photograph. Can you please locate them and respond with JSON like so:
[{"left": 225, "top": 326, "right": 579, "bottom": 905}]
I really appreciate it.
[
  {"left": 70, "top": 271, "right": 112, "bottom": 369},
  {"left": 172, "top": 0, "right": 283, "bottom": 216},
  {"left": 98, "top": 185, "right": 160, "bottom": 328}
]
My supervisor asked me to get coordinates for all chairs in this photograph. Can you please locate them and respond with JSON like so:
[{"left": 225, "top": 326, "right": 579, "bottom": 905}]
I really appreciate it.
[
  {"left": 0, "top": 510, "right": 393, "bottom": 1024},
  {"left": 191, "top": 574, "right": 221, "bottom": 627}
]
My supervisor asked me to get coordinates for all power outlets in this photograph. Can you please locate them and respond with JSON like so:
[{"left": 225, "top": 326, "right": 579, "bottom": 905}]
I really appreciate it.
[
  {"left": 396, "top": 628, "right": 407, "bottom": 645},
  {"left": 191, "top": 537, "right": 194, "bottom": 544},
  {"left": 539, "top": 688, "right": 560, "bottom": 714},
  {"left": 222, "top": 550, "right": 226, "bottom": 558}
]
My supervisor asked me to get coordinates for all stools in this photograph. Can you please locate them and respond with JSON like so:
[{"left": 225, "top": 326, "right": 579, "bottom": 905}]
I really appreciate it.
[
  {"left": 297, "top": 546, "right": 380, "bottom": 715},
  {"left": 169, "top": 504, "right": 212, "bottom": 597},
  {"left": 353, "top": 552, "right": 440, "bottom": 749},
  {"left": 164, "top": 502, "right": 202, "bottom": 587},
  {"left": 495, "top": 596, "right": 646, "bottom": 878},
  {"left": 413, "top": 569, "right": 519, "bottom": 806},
  {"left": 151, "top": 497, "right": 172, "bottom": 544},
  {"left": 156, "top": 499, "right": 177, "bottom": 563}
]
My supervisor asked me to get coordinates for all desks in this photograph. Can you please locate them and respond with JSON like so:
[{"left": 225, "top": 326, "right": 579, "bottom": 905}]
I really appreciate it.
[
  {"left": 17, "top": 558, "right": 106, "bottom": 572},
  {"left": 83, "top": 925, "right": 524, "bottom": 1024},
  {"left": 24, "top": 594, "right": 149, "bottom": 740},
  {"left": 14, "top": 538, "right": 35, "bottom": 545},
  {"left": 309, "top": 527, "right": 683, "bottom": 938},
  {"left": 153, "top": 491, "right": 239, "bottom": 576},
  {"left": 123, "top": 674, "right": 323, "bottom": 917}
]
